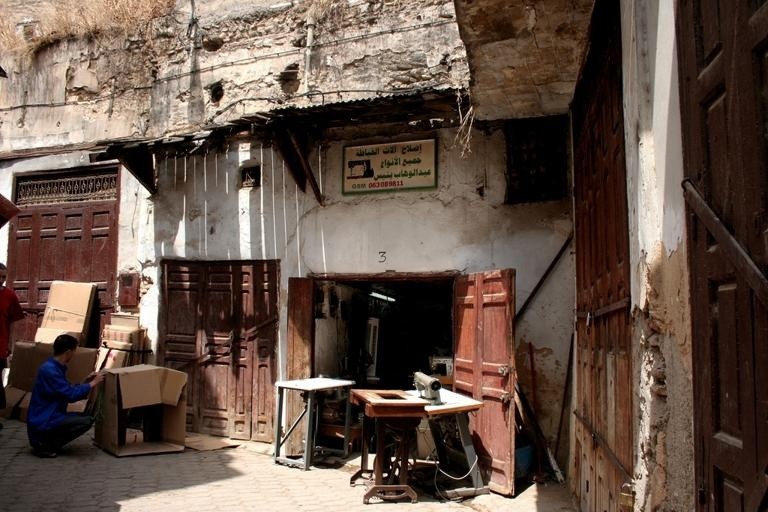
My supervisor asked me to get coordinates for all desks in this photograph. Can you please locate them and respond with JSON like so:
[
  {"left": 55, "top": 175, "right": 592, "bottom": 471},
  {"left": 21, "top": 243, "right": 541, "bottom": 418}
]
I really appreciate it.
[{"left": 273, "top": 377, "right": 357, "bottom": 471}]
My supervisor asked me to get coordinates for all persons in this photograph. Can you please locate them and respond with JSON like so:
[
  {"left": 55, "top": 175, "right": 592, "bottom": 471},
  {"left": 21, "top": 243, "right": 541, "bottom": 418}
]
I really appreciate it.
[
  {"left": 27, "top": 335, "right": 106, "bottom": 458},
  {"left": 0, "top": 263, "right": 26, "bottom": 428}
]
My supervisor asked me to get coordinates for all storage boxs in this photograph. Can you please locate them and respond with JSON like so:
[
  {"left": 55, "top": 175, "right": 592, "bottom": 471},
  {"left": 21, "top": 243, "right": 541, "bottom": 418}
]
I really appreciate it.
[{"left": 91, "top": 362, "right": 189, "bottom": 460}]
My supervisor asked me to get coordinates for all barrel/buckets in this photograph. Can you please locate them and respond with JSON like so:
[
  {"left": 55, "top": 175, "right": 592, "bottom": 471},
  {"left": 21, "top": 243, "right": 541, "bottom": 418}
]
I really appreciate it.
[{"left": 516, "top": 445, "right": 533, "bottom": 478}]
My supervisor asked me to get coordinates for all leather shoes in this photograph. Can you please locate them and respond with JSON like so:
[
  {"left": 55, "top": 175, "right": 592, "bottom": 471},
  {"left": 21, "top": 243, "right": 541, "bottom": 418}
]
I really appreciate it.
[{"left": 31, "top": 442, "right": 57, "bottom": 457}]
